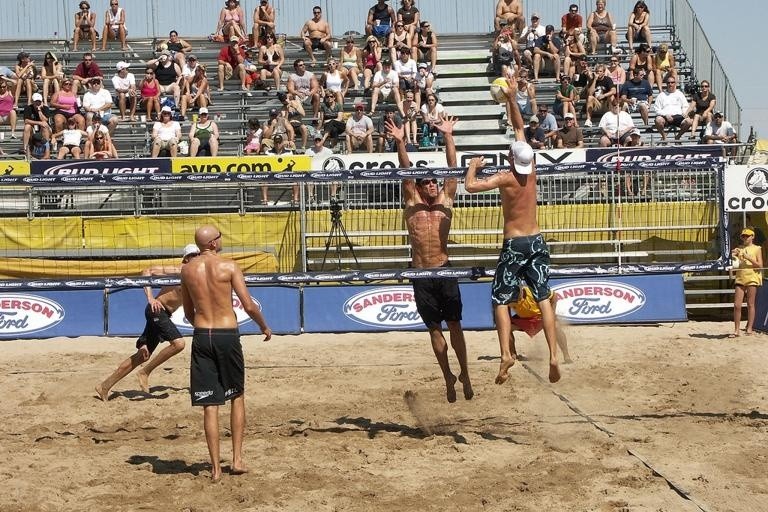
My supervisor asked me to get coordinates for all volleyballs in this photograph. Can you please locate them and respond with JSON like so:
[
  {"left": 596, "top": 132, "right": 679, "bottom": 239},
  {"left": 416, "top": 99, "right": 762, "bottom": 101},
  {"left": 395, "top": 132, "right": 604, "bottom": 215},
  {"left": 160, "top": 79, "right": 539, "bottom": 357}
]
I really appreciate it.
[{"left": 490, "top": 77, "right": 509, "bottom": 103}]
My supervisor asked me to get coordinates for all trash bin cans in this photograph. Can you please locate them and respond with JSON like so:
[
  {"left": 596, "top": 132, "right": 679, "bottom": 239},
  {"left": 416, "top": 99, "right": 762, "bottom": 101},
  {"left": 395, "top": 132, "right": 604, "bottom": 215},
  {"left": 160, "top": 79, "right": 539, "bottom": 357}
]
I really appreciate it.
[{"left": 752, "top": 279, "right": 768, "bottom": 332}]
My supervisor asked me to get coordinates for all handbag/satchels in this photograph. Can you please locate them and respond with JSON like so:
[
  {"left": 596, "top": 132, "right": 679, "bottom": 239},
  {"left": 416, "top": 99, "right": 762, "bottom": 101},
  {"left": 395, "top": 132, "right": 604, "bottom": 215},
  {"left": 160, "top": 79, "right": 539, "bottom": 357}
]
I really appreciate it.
[
  {"left": 179, "top": 141, "right": 189, "bottom": 155},
  {"left": 405, "top": 143, "right": 418, "bottom": 152},
  {"left": 254, "top": 79, "right": 271, "bottom": 90}
]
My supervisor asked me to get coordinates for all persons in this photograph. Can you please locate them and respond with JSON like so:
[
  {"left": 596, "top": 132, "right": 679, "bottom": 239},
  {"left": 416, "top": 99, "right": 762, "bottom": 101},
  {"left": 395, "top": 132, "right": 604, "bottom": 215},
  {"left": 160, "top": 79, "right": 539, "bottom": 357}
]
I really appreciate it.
[
  {"left": 383, "top": 111, "right": 475, "bottom": 403},
  {"left": 485, "top": 0, "right": 739, "bottom": 199},
  {"left": 463, "top": 74, "right": 561, "bottom": 385},
  {"left": 730, "top": 212, "right": 764, "bottom": 338},
  {"left": 94, "top": 243, "right": 201, "bottom": 403},
  {"left": 507, "top": 282, "right": 576, "bottom": 365},
  {"left": 179, "top": 222, "right": 272, "bottom": 483},
  {"left": 0, "top": 0, "right": 457, "bottom": 210}
]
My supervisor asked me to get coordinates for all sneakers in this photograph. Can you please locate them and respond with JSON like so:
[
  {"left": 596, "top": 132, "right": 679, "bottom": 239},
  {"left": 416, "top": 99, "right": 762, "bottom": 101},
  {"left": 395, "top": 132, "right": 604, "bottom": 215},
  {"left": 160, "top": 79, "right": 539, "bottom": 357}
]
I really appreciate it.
[
  {"left": 10, "top": 134, "right": 17, "bottom": 138},
  {"left": 646, "top": 128, "right": 652, "bottom": 133},
  {"left": 585, "top": 119, "right": 592, "bottom": 127},
  {"left": 13, "top": 105, "right": 18, "bottom": 109},
  {"left": 240, "top": 85, "right": 253, "bottom": 97}
]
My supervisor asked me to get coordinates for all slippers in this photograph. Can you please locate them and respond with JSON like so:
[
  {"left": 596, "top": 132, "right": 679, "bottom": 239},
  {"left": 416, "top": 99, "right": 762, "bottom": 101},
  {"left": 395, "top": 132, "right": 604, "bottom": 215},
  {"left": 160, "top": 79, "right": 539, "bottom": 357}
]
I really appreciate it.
[{"left": 729, "top": 333, "right": 753, "bottom": 338}]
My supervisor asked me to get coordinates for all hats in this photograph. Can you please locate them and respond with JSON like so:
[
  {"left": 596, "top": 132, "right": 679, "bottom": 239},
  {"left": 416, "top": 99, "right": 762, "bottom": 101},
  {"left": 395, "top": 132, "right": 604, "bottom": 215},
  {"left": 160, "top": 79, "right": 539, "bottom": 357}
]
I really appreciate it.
[
  {"left": 714, "top": 110, "right": 723, "bottom": 117},
  {"left": 32, "top": 134, "right": 47, "bottom": 145},
  {"left": 161, "top": 49, "right": 170, "bottom": 56},
  {"left": 181, "top": 244, "right": 200, "bottom": 264},
  {"left": 90, "top": 77, "right": 100, "bottom": 83},
  {"left": 740, "top": 229, "right": 755, "bottom": 237},
  {"left": 270, "top": 108, "right": 278, "bottom": 116},
  {"left": 279, "top": 94, "right": 286, "bottom": 101},
  {"left": 189, "top": 54, "right": 198, "bottom": 60},
  {"left": 314, "top": 133, "right": 322, "bottom": 140},
  {"left": 499, "top": 12, "right": 554, "bottom": 31},
  {"left": 225, "top": 0, "right": 239, "bottom": 5},
  {"left": 199, "top": 107, "right": 209, "bottom": 115},
  {"left": 418, "top": 63, "right": 427, "bottom": 68},
  {"left": 355, "top": 103, "right": 364, "bottom": 110},
  {"left": 116, "top": 61, "right": 131, "bottom": 71},
  {"left": 529, "top": 115, "right": 539, "bottom": 123},
  {"left": 510, "top": 141, "right": 534, "bottom": 175},
  {"left": 274, "top": 135, "right": 282, "bottom": 142},
  {"left": 230, "top": 36, "right": 239, "bottom": 43},
  {"left": 160, "top": 106, "right": 171, "bottom": 113},
  {"left": 564, "top": 113, "right": 573, "bottom": 119},
  {"left": 31, "top": 93, "right": 42, "bottom": 102},
  {"left": 92, "top": 113, "right": 101, "bottom": 119},
  {"left": 17, "top": 52, "right": 29, "bottom": 59},
  {"left": 629, "top": 128, "right": 641, "bottom": 136},
  {"left": 68, "top": 117, "right": 76, "bottom": 122},
  {"left": 559, "top": 73, "right": 571, "bottom": 83}
]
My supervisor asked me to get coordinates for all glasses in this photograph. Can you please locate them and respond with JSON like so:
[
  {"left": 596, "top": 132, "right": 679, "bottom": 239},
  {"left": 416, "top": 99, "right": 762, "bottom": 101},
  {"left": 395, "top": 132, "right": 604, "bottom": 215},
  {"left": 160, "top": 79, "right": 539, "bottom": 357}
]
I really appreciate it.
[
  {"left": 423, "top": 179, "right": 437, "bottom": 185},
  {"left": 0, "top": 86, "right": 6, "bottom": 88},
  {"left": 397, "top": 25, "right": 430, "bottom": 55},
  {"left": 188, "top": 253, "right": 200, "bottom": 258},
  {"left": 266, "top": 36, "right": 274, "bottom": 39},
  {"left": 208, "top": 232, "right": 221, "bottom": 244},
  {"left": 96, "top": 137, "right": 103, "bottom": 140}
]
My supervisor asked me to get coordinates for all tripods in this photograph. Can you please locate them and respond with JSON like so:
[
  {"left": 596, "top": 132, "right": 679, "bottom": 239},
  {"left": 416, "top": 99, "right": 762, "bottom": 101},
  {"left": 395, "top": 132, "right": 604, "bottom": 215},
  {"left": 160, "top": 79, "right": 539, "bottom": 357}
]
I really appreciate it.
[{"left": 316, "top": 220, "right": 369, "bottom": 285}]
